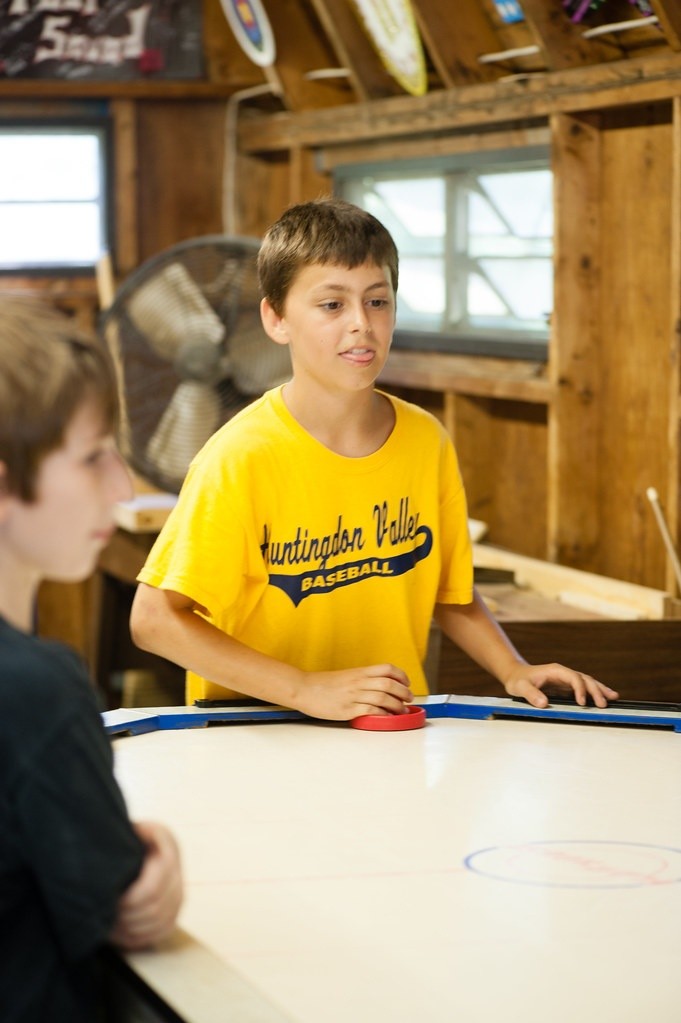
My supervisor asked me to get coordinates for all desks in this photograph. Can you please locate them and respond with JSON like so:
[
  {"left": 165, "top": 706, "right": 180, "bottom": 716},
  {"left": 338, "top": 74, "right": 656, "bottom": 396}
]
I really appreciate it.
[
  {"left": 100, "top": 695, "right": 680, "bottom": 1023},
  {"left": 34, "top": 527, "right": 680, "bottom": 709}
]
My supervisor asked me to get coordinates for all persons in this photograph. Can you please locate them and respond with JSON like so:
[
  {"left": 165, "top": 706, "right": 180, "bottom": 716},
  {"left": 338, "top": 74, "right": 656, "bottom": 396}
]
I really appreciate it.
[
  {"left": 130, "top": 200, "right": 617, "bottom": 726},
  {"left": 0, "top": 281, "right": 184, "bottom": 1023}
]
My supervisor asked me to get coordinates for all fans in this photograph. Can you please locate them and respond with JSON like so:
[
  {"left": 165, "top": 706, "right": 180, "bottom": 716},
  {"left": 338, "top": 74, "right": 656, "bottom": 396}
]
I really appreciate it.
[{"left": 93, "top": 234, "right": 293, "bottom": 499}]
